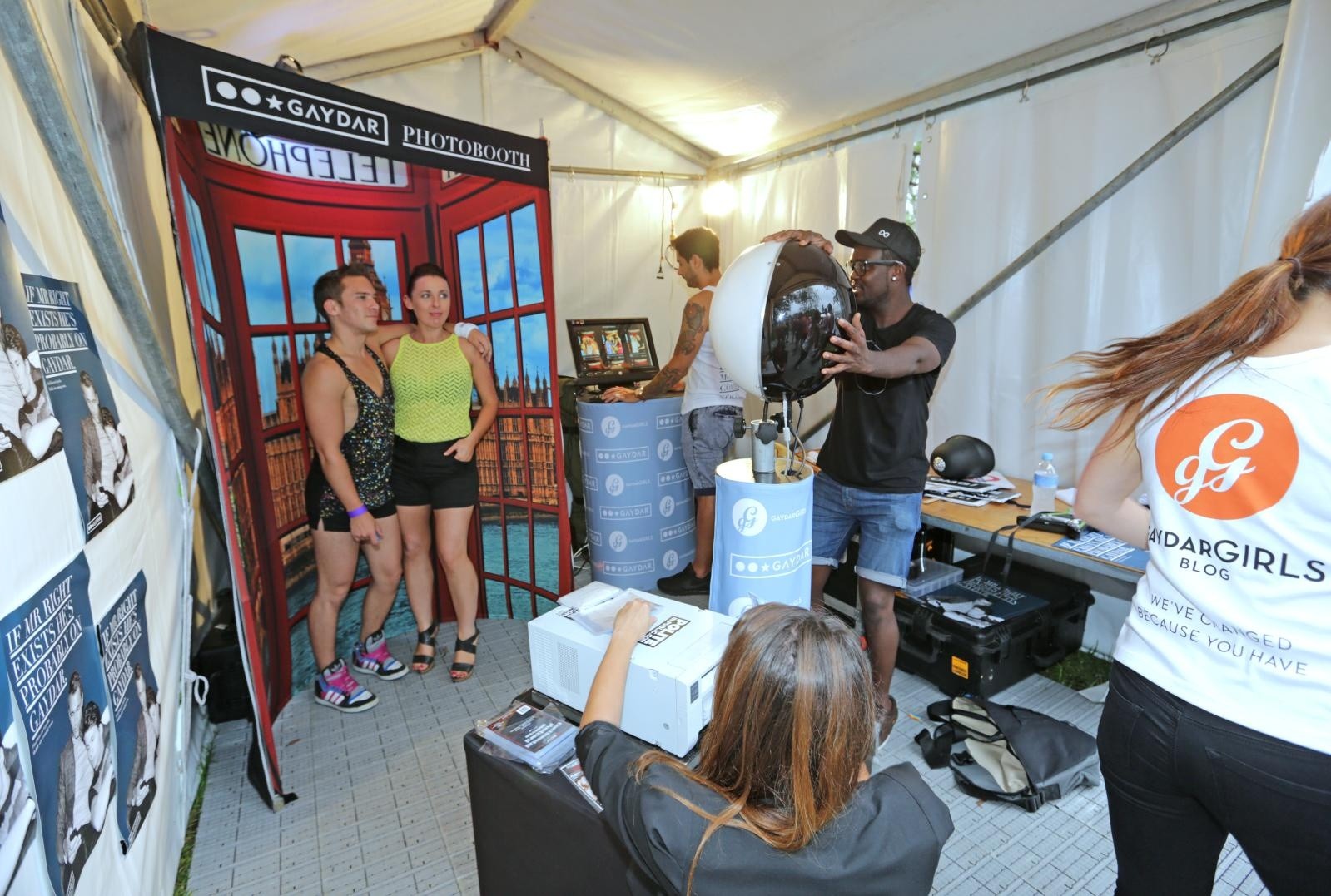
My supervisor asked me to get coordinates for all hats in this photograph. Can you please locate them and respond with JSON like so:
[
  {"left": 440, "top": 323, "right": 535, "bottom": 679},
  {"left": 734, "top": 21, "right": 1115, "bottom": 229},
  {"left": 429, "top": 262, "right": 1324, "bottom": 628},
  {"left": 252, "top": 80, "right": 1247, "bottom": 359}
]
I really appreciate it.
[{"left": 834, "top": 218, "right": 921, "bottom": 270}]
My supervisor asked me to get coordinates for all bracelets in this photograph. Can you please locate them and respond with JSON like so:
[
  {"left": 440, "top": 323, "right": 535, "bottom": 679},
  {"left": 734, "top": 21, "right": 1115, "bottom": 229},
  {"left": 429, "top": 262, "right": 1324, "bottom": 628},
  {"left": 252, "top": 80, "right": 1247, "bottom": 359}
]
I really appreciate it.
[
  {"left": 347, "top": 504, "right": 368, "bottom": 518},
  {"left": 454, "top": 322, "right": 479, "bottom": 342}
]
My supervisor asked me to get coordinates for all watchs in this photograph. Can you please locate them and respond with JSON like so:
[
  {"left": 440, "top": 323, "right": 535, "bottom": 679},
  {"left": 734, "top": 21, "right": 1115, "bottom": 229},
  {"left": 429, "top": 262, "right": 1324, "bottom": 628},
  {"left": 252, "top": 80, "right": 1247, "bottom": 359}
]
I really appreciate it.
[{"left": 635, "top": 387, "right": 646, "bottom": 400}]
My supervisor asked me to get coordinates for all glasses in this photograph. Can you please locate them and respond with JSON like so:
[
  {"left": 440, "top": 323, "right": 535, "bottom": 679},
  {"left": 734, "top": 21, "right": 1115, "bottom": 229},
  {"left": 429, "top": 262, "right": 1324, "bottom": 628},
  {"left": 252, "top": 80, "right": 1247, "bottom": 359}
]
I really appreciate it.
[{"left": 846, "top": 259, "right": 905, "bottom": 277}]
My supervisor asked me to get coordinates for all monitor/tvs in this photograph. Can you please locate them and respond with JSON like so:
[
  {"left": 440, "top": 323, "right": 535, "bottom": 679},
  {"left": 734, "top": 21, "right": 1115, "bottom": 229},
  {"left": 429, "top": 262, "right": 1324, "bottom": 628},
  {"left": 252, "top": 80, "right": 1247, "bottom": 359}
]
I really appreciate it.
[{"left": 565, "top": 317, "right": 660, "bottom": 394}]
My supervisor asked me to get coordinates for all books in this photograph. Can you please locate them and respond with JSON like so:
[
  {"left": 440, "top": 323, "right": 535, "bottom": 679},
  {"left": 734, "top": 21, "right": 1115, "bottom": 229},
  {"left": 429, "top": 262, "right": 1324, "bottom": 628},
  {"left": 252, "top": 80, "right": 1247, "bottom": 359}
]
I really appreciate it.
[
  {"left": 924, "top": 476, "right": 1021, "bottom": 507},
  {"left": 482, "top": 703, "right": 605, "bottom": 813}
]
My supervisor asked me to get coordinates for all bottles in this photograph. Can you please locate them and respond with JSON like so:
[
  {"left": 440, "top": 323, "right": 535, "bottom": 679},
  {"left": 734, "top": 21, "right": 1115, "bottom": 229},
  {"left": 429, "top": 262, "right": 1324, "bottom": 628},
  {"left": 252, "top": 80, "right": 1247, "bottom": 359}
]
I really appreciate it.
[{"left": 1029, "top": 452, "right": 1059, "bottom": 517}]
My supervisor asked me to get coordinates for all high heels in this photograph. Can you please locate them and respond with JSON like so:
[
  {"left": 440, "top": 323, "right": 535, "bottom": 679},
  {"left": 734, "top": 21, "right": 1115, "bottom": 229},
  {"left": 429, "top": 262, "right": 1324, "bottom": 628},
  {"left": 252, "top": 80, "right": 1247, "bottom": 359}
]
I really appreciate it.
[
  {"left": 411, "top": 621, "right": 438, "bottom": 674},
  {"left": 450, "top": 625, "right": 480, "bottom": 683}
]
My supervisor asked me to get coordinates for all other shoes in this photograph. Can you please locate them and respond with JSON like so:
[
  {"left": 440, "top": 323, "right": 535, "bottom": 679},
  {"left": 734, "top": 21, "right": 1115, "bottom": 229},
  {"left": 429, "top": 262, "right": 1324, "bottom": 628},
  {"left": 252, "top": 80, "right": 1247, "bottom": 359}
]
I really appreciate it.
[
  {"left": 657, "top": 562, "right": 711, "bottom": 596},
  {"left": 874, "top": 693, "right": 897, "bottom": 747}
]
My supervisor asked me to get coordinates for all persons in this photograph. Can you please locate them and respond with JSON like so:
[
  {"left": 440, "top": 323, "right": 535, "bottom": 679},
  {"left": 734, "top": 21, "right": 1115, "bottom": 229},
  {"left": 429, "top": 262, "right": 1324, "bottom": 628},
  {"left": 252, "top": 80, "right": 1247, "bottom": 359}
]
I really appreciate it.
[
  {"left": 77, "top": 372, "right": 135, "bottom": 542},
  {"left": 54, "top": 673, "right": 117, "bottom": 896},
  {"left": 382, "top": 263, "right": 500, "bottom": 682},
  {"left": 1033, "top": 188, "right": 1330, "bottom": 896},
  {"left": 125, "top": 663, "right": 161, "bottom": 840},
  {"left": 781, "top": 302, "right": 835, "bottom": 369},
  {"left": 0, "top": 722, "right": 37, "bottom": 896},
  {"left": 572, "top": 599, "right": 955, "bottom": 896},
  {"left": 300, "top": 263, "right": 492, "bottom": 712},
  {"left": 598, "top": 227, "right": 747, "bottom": 594},
  {"left": 0, "top": 308, "right": 65, "bottom": 483},
  {"left": 760, "top": 217, "right": 956, "bottom": 746}
]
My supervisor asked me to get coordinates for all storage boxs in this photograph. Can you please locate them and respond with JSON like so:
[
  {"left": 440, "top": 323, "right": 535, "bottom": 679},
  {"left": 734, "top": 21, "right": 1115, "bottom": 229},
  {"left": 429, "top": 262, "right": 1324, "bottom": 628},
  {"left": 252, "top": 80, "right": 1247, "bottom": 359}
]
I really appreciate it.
[{"left": 527, "top": 589, "right": 740, "bottom": 758}]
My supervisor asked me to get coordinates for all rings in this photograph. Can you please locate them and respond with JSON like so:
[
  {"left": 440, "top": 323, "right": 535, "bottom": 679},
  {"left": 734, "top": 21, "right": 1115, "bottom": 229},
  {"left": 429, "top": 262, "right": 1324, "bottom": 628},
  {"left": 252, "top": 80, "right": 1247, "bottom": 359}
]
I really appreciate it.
[{"left": 615, "top": 393, "right": 620, "bottom": 397}]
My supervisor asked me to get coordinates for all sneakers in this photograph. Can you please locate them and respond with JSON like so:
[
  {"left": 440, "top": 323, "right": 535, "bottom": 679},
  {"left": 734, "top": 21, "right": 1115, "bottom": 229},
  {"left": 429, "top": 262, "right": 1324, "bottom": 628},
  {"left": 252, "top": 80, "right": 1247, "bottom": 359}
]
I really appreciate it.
[
  {"left": 314, "top": 657, "right": 380, "bottom": 713},
  {"left": 352, "top": 629, "right": 409, "bottom": 680}
]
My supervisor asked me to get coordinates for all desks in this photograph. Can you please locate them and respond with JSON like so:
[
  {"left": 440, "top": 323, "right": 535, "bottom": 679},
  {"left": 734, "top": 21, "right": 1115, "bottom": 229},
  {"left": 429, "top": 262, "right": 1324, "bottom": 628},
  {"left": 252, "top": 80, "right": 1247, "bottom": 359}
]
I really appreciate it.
[{"left": 795, "top": 448, "right": 1147, "bottom": 604}]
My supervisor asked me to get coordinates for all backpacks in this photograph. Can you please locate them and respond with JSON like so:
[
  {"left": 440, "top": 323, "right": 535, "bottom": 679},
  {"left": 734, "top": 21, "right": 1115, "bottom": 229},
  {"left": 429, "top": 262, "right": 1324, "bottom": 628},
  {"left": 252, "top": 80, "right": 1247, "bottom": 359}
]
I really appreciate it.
[{"left": 912, "top": 689, "right": 1104, "bottom": 813}]
